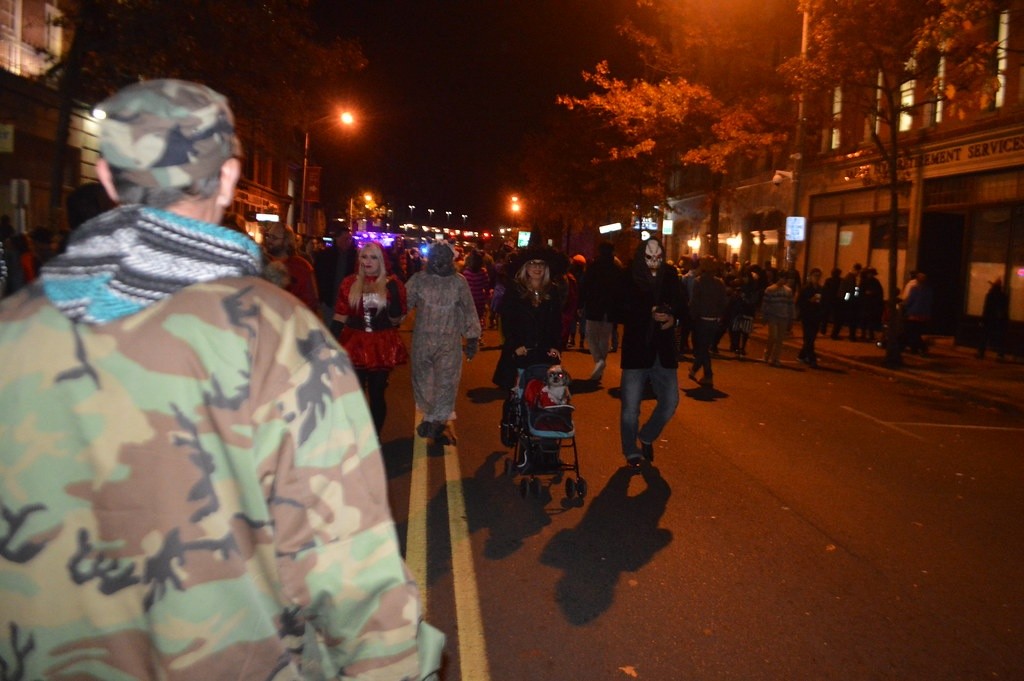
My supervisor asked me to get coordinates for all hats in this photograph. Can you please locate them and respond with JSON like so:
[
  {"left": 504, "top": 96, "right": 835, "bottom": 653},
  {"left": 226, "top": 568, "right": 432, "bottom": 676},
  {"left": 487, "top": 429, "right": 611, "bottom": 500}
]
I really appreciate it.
[{"left": 99, "top": 79, "right": 244, "bottom": 188}]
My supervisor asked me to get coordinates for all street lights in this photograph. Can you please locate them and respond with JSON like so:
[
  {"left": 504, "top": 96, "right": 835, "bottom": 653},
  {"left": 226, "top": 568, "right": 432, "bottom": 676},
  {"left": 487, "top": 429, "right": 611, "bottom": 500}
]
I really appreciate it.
[
  {"left": 350, "top": 196, "right": 373, "bottom": 234},
  {"left": 428, "top": 209, "right": 435, "bottom": 225},
  {"left": 408, "top": 205, "right": 415, "bottom": 222},
  {"left": 446, "top": 211, "right": 453, "bottom": 228},
  {"left": 462, "top": 214, "right": 469, "bottom": 226},
  {"left": 388, "top": 209, "right": 395, "bottom": 230},
  {"left": 294, "top": 112, "right": 353, "bottom": 246},
  {"left": 510, "top": 201, "right": 519, "bottom": 227}
]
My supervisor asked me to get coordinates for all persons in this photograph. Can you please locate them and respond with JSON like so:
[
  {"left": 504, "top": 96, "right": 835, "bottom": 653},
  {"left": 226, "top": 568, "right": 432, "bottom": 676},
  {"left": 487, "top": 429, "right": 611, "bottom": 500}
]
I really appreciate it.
[
  {"left": 976, "top": 278, "right": 1008, "bottom": 361},
  {"left": 0, "top": 183, "right": 118, "bottom": 299},
  {"left": 225, "top": 219, "right": 492, "bottom": 448},
  {"left": 494, "top": 237, "right": 882, "bottom": 387},
  {"left": 616, "top": 238, "right": 687, "bottom": 470},
  {"left": 496, "top": 251, "right": 565, "bottom": 470},
  {"left": 0, "top": 80, "right": 444, "bottom": 681},
  {"left": 898, "top": 270, "right": 932, "bottom": 356}
]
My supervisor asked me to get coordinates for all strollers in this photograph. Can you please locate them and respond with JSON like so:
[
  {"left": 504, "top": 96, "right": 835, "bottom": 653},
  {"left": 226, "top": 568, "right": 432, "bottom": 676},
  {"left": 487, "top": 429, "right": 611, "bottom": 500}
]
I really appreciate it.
[{"left": 511, "top": 345, "right": 589, "bottom": 500}]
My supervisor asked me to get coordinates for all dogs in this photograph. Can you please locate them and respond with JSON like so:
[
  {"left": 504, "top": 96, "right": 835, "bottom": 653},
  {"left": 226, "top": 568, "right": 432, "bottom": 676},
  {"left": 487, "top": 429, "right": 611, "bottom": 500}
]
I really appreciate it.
[{"left": 523, "top": 364, "right": 573, "bottom": 412}]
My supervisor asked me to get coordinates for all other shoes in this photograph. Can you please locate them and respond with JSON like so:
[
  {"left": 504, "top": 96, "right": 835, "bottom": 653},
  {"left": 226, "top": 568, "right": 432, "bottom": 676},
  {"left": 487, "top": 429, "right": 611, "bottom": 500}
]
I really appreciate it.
[
  {"left": 432, "top": 424, "right": 444, "bottom": 437},
  {"left": 627, "top": 457, "right": 641, "bottom": 473},
  {"left": 637, "top": 432, "right": 653, "bottom": 461},
  {"left": 590, "top": 361, "right": 607, "bottom": 382},
  {"left": 416, "top": 421, "right": 432, "bottom": 437}
]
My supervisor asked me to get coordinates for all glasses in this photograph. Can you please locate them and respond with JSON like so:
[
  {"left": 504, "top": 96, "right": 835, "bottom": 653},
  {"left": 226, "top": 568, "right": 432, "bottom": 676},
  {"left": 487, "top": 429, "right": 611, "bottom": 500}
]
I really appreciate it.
[{"left": 526, "top": 261, "right": 545, "bottom": 270}]
